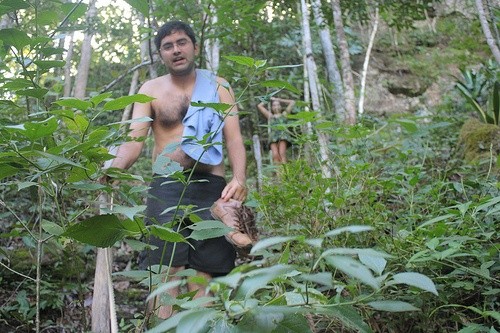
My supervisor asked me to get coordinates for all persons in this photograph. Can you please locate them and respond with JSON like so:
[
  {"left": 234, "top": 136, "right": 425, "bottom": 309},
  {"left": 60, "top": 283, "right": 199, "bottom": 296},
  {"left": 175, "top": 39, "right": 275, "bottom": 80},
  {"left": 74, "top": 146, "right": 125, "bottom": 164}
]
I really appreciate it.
[
  {"left": 258, "top": 97, "right": 296, "bottom": 167},
  {"left": 98, "top": 21, "right": 249, "bottom": 332}
]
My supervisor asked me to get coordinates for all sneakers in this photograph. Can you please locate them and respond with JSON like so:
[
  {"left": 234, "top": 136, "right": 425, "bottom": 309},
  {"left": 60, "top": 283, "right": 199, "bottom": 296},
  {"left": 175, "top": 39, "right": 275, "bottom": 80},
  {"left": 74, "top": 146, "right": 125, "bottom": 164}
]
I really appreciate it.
[
  {"left": 234, "top": 205, "right": 265, "bottom": 261},
  {"left": 210, "top": 197, "right": 252, "bottom": 246}
]
